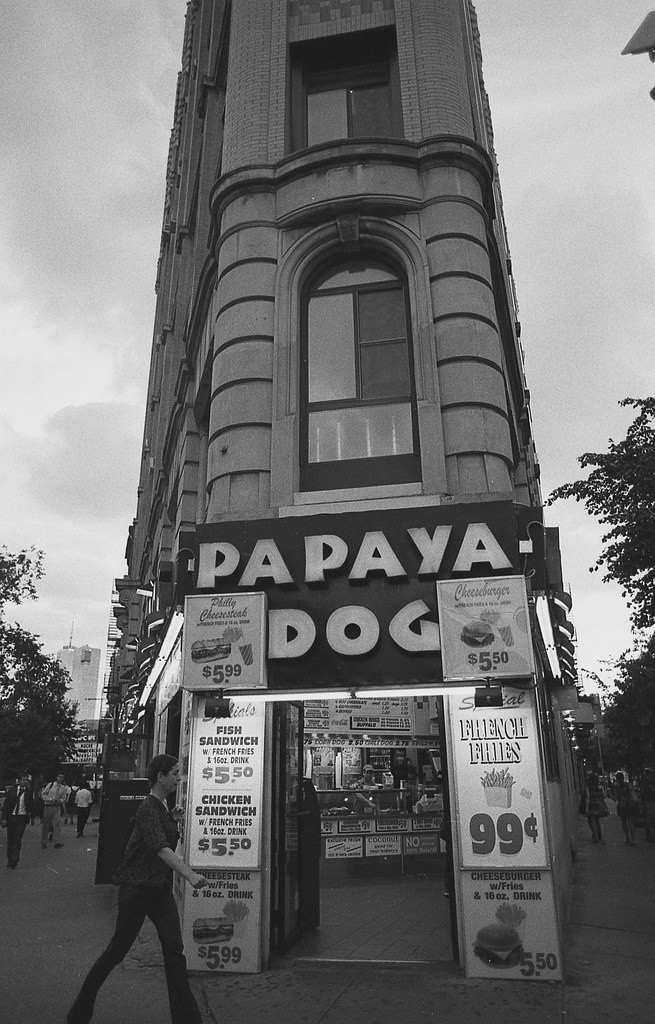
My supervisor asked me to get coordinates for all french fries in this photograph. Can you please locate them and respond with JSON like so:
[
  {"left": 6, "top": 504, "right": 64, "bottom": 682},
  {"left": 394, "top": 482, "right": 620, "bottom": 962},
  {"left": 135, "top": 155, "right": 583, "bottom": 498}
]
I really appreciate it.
[
  {"left": 479, "top": 767, "right": 516, "bottom": 788},
  {"left": 222, "top": 628, "right": 242, "bottom": 643},
  {"left": 494, "top": 903, "right": 527, "bottom": 926},
  {"left": 223, "top": 900, "right": 250, "bottom": 922}
]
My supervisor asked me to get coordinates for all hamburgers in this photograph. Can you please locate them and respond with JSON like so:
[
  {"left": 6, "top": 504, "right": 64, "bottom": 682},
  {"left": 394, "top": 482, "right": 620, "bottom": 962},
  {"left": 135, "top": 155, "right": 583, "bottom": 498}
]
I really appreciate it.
[
  {"left": 193, "top": 917, "right": 234, "bottom": 944},
  {"left": 191, "top": 637, "right": 231, "bottom": 663},
  {"left": 460, "top": 620, "right": 495, "bottom": 648},
  {"left": 473, "top": 923, "right": 524, "bottom": 968}
]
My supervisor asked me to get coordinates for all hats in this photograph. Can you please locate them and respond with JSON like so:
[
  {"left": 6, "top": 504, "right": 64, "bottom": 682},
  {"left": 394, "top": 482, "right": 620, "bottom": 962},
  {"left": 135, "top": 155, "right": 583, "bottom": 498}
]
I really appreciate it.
[{"left": 363, "top": 764, "right": 375, "bottom": 772}]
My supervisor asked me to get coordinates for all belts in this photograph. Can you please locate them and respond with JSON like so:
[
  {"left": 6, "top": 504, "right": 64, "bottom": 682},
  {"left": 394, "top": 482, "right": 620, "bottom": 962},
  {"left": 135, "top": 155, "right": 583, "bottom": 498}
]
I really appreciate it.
[{"left": 44, "top": 804, "right": 59, "bottom": 807}]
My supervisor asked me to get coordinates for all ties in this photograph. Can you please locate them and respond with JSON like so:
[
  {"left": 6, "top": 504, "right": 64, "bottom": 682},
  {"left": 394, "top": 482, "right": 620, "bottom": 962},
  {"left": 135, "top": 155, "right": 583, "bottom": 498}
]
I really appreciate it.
[{"left": 16, "top": 789, "right": 24, "bottom": 815}]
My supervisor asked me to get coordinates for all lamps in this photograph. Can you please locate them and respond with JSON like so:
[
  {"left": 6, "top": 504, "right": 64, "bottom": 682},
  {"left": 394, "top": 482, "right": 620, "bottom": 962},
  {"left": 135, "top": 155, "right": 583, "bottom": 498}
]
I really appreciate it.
[
  {"left": 204, "top": 690, "right": 235, "bottom": 718},
  {"left": 474, "top": 678, "right": 503, "bottom": 707}
]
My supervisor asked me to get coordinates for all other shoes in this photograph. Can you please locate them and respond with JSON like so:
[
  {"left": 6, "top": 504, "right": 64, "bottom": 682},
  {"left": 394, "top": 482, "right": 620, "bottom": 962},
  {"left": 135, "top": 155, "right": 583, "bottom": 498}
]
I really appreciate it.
[
  {"left": 42, "top": 845, "right": 47, "bottom": 849},
  {"left": 54, "top": 843, "right": 65, "bottom": 847}
]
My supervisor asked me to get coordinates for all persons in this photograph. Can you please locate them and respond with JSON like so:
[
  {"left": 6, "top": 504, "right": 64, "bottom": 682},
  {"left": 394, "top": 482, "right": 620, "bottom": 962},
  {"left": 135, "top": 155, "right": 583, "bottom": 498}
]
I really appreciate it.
[
  {"left": 353, "top": 765, "right": 379, "bottom": 813},
  {"left": 75, "top": 783, "right": 93, "bottom": 838},
  {"left": 41, "top": 773, "right": 66, "bottom": 849},
  {"left": 609, "top": 772, "right": 638, "bottom": 845},
  {"left": 37, "top": 774, "right": 91, "bottom": 825},
  {"left": 1, "top": 772, "right": 35, "bottom": 868},
  {"left": 584, "top": 774, "right": 607, "bottom": 843},
  {"left": 66, "top": 754, "right": 210, "bottom": 1024},
  {"left": 298, "top": 778, "right": 323, "bottom": 928}
]
